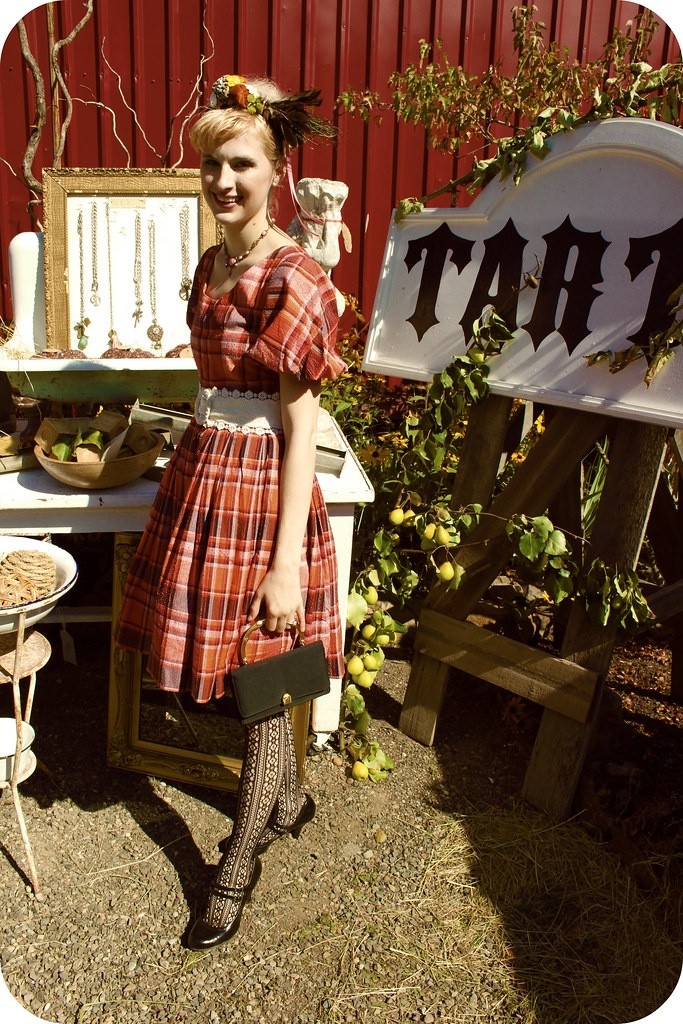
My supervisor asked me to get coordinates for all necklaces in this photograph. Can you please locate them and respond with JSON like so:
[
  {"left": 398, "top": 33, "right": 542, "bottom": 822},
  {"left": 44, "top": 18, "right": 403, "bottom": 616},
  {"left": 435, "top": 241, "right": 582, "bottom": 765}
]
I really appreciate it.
[
  {"left": 77, "top": 201, "right": 190, "bottom": 351},
  {"left": 221, "top": 224, "right": 271, "bottom": 276}
]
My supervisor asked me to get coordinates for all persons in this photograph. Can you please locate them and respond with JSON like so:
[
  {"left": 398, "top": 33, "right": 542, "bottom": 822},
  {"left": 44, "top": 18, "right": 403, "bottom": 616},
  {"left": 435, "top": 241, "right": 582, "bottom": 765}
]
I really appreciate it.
[{"left": 112, "top": 73, "right": 350, "bottom": 951}]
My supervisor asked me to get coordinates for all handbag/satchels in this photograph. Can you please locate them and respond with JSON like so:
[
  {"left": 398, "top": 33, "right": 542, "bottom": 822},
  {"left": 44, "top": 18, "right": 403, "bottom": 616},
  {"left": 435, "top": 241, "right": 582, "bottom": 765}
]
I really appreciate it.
[{"left": 229, "top": 619, "right": 331, "bottom": 727}]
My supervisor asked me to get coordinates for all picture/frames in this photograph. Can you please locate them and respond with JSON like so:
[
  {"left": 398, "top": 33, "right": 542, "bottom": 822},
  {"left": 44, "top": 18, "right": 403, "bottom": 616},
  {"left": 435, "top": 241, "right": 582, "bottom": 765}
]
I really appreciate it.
[{"left": 41, "top": 166, "right": 222, "bottom": 356}]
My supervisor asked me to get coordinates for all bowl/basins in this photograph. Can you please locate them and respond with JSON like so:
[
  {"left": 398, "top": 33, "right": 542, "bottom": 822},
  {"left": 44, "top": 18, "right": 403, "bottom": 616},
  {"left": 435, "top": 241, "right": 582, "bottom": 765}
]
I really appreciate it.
[
  {"left": 0, "top": 536, "right": 78, "bottom": 634},
  {"left": 33, "top": 431, "right": 165, "bottom": 489},
  {"left": 0, "top": 717, "right": 36, "bottom": 781}
]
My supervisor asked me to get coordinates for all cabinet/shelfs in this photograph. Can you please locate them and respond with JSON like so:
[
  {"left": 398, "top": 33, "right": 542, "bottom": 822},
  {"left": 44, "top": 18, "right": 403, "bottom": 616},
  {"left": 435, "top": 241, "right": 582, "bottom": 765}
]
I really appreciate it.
[{"left": 0, "top": 534, "right": 79, "bottom": 902}]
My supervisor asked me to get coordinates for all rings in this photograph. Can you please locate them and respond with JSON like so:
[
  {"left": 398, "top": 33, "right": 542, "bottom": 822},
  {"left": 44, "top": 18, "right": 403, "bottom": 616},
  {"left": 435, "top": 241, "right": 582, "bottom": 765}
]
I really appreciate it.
[{"left": 285, "top": 619, "right": 298, "bottom": 627}]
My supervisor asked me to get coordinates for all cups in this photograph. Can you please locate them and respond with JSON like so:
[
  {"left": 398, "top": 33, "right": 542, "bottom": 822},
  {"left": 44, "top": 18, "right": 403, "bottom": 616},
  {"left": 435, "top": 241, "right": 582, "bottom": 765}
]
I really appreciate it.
[{"left": 12, "top": 395, "right": 49, "bottom": 455}]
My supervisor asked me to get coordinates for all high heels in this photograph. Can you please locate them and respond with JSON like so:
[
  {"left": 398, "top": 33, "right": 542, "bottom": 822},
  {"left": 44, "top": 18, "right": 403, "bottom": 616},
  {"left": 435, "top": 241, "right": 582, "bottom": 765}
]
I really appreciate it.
[
  {"left": 214, "top": 794, "right": 316, "bottom": 857},
  {"left": 187, "top": 852, "right": 280, "bottom": 951}
]
